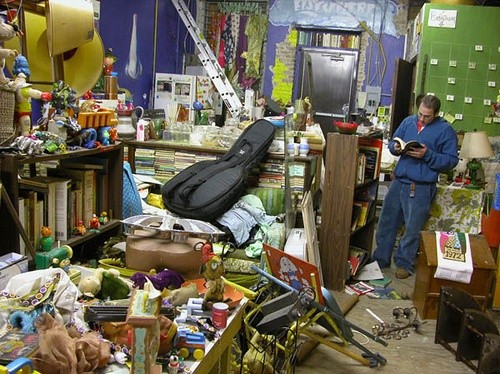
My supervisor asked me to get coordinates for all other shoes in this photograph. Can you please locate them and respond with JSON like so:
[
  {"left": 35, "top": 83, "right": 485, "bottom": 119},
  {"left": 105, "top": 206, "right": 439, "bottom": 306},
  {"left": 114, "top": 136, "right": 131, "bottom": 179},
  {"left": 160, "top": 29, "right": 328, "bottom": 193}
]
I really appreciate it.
[{"left": 395, "top": 266, "right": 410, "bottom": 279}]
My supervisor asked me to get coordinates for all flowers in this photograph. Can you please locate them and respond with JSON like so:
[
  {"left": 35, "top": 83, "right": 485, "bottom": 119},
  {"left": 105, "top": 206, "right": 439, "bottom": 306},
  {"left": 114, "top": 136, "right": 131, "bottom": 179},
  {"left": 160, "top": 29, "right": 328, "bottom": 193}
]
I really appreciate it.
[{"left": 492, "top": 102, "right": 500, "bottom": 117}]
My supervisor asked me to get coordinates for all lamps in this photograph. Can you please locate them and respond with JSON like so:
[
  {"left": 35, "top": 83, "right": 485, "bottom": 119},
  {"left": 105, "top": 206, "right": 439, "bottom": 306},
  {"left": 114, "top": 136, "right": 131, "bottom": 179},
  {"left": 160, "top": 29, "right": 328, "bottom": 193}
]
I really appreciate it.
[{"left": 460, "top": 130, "right": 496, "bottom": 190}]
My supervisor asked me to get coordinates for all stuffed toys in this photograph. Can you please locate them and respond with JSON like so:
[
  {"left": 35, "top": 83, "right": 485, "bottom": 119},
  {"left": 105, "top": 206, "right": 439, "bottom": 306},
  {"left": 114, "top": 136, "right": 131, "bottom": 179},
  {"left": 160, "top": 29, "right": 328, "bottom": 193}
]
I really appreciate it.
[
  {"left": 0, "top": 18, "right": 19, "bottom": 84},
  {"left": 200, "top": 244, "right": 226, "bottom": 311},
  {"left": 78, "top": 267, "right": 130, "bottom": 302},
  {"left": 130, "top": 269, "right": 185, "bottom": 292}
]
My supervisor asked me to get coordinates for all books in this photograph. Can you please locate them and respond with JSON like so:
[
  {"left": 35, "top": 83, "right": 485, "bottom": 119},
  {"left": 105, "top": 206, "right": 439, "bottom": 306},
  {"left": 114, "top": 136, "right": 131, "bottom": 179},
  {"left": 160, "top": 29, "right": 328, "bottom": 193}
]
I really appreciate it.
[
  {"left": 343, "top": 138, "right": 403, "bottom": 301},
  {"left": 124, "top": 130, "right": 325, "bottom": 211},
  {"left": 15, "top": 160, "right": 110, "bottom": 261},
  {"left": 392, "top": 136, "right": 424, "bottom": 155}
]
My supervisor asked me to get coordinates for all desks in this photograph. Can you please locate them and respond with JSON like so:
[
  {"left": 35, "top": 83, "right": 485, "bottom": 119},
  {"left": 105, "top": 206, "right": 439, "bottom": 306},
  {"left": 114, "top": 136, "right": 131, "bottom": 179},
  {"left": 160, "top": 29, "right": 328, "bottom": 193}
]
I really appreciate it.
[{"left": 436, "top": 185, "right": 488, "bottom": 234}]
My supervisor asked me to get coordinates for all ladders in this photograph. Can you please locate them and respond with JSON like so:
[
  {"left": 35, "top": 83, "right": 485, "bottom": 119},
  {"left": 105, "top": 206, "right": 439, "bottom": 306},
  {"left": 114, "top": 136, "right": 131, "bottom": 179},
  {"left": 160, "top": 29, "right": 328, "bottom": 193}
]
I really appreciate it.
[{"left": 163, "top": 0, "right": 247, "bottom": 119}]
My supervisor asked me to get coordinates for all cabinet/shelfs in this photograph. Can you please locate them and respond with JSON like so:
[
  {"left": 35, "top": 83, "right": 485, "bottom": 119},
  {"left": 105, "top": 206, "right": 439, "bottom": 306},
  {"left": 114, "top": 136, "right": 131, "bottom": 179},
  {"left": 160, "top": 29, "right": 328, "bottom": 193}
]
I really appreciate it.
[
  {"left": 155, "top": 73, "right": 196, "bottom": 125},
  {"left": 411, "top": 230, "right": 497, "bottom": 320},
  {"left": 2, "top": 132, "right": 381, "bottom": 292}
]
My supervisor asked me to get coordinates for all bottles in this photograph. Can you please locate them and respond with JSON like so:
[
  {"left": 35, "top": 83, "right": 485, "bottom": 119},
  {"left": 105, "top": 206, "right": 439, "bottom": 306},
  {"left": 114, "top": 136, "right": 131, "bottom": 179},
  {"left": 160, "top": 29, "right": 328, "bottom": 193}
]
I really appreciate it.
[
  {"left": 103, "top": 75, "right": 119, "bottom": 100},
  {"left": 286, "top": 137, "right": 296, "bottom": 157},
  {"left": 299, "top": 137, "right": 309, "bottom": 156}
]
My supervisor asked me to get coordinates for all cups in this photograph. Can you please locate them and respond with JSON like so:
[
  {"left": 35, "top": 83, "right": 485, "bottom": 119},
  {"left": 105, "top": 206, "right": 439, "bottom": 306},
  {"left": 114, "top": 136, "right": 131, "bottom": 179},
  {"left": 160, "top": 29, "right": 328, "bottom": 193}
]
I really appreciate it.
[{"left": 211, "top": 302, "right": 230, "bottom": 330}]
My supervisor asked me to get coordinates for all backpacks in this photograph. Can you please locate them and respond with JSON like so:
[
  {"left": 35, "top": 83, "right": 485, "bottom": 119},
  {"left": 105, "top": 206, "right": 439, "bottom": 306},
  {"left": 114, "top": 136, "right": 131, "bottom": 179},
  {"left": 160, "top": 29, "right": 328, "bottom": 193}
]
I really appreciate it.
[{"left": 162, "top": 118, "right": 275, "bottom": 221}]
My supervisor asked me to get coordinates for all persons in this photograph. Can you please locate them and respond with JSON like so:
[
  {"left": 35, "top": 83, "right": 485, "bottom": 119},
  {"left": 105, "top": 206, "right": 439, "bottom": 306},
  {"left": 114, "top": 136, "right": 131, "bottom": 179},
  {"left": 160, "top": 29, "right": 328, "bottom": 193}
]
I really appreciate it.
[{"left": 367, "top": 95, "right": 459, "bottom": 279}]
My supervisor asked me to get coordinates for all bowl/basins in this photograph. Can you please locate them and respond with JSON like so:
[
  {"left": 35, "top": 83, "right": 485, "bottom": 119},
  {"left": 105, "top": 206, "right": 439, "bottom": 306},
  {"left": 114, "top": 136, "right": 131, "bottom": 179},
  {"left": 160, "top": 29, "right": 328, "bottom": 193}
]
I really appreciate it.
[{"left": 336, "top": 122, "right": 359, "bottom": 133}]
